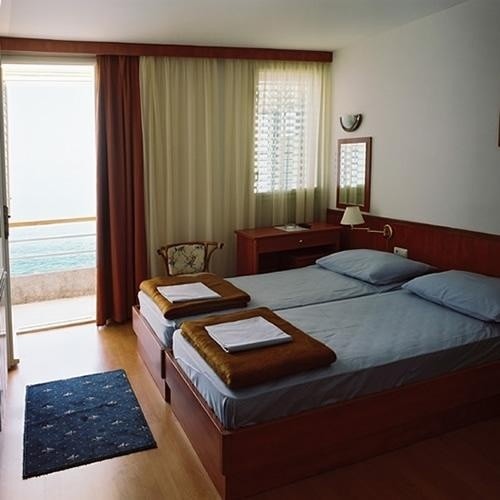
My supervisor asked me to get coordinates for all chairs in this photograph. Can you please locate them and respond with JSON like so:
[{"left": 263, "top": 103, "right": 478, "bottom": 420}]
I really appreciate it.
[{"left": 157, "top": 241, "right": 225, "bottom": 277}]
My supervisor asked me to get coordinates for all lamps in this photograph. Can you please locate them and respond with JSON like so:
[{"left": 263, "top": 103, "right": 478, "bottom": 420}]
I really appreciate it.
[
  {"left": 341, "top": 206, "right": 393, "bottom": 239},
  {"left": 339, "top": 113, "right": 363, "bottom": 132}
]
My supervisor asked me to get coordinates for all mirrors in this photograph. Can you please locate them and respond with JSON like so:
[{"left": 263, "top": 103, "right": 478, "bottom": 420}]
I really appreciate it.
[{"left": 337, "top": 136, "right": 373, "bottom": 212}]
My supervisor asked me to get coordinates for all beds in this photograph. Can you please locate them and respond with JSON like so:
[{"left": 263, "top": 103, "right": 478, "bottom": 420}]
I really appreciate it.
[{"left": 132, "top": 211, "right": 500, "bottom": 500}]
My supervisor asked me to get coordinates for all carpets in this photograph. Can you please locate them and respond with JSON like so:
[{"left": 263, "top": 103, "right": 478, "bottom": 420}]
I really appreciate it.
[{"left": 22, "top": 368, "right": 159, "bottom": 480}]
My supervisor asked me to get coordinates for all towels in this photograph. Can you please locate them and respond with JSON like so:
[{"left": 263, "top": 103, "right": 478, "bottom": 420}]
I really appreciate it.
[
  {"left": 206, "top": 316, "right": 292, "bottom": 353},
  {"left": 156, "top": 281, "right": 222, "bottom": 304}
]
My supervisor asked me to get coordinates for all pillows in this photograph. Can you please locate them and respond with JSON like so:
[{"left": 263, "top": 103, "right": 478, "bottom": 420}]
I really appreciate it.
[
  {"left": 315, "top": 248, "right": 440, "bottom": 285},
  {"left": 400, "top": 270, "right": 500, "bottom": 322}
]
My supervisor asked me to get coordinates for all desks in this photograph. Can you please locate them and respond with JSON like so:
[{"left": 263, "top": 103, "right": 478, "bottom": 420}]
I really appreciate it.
[{"left": 235, "top": 222, "right": 343, "bottom": 276}]
